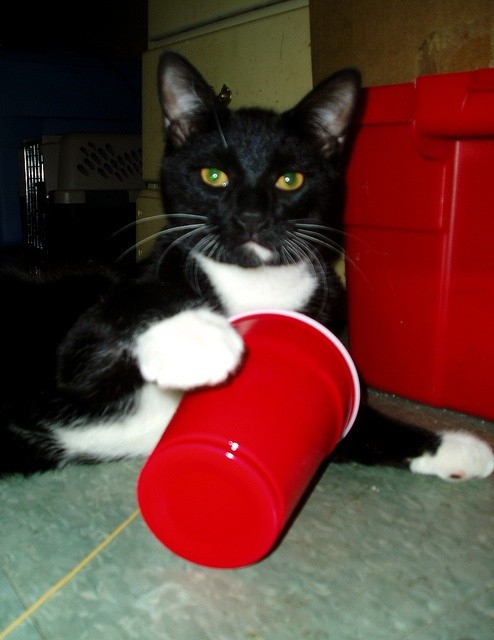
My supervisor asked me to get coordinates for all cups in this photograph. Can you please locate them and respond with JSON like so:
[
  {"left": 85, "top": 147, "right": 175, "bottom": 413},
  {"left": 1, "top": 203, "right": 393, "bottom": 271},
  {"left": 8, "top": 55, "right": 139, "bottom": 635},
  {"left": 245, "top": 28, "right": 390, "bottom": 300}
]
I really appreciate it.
[{"left": 136, "top": 313, "right": 362, "bottom": 568}]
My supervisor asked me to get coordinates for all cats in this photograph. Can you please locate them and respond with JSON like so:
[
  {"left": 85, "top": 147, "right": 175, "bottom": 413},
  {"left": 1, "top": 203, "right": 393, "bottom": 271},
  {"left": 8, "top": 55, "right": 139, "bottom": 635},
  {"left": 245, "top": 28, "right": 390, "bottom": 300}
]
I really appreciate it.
[{"left": 0, "top": 52, "right": 493, "bottom": 480}]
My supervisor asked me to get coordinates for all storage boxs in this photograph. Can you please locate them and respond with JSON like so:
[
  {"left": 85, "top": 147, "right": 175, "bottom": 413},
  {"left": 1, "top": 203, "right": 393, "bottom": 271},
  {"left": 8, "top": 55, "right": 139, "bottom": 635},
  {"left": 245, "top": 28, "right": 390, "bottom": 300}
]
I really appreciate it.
[{"left": 336, "top": 66, "right": 494, "bottom": 422}]
